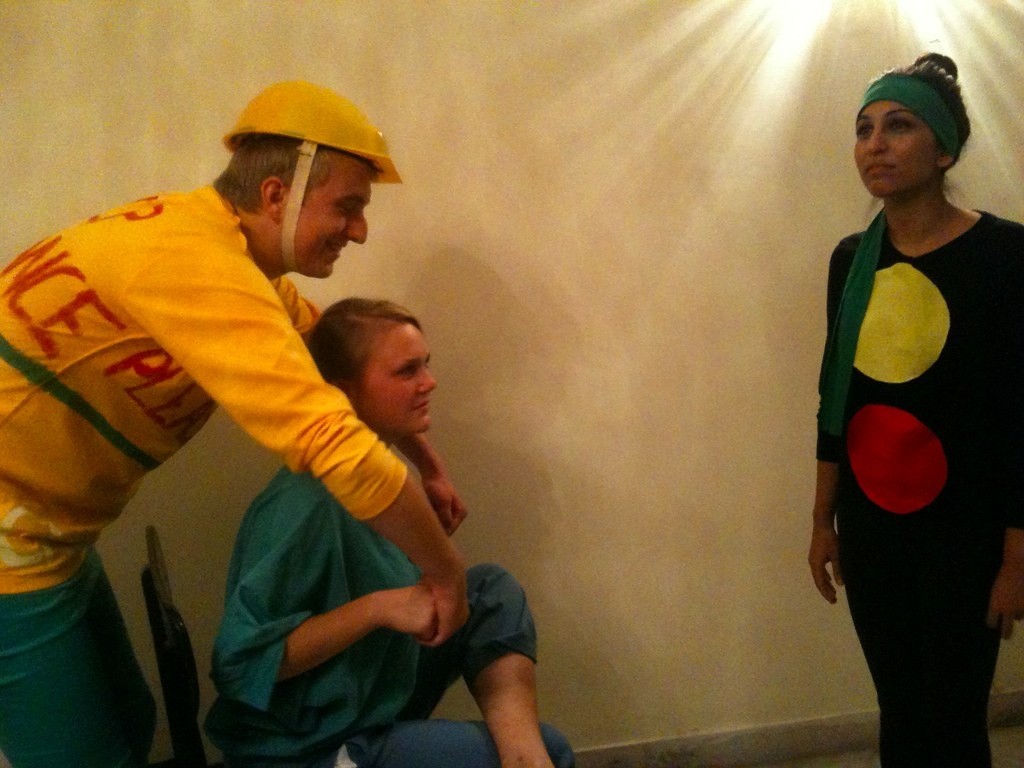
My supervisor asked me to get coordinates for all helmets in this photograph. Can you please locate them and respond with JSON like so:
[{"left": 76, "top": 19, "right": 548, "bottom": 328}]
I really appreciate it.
[{"left": 224, "top": 81, "right": 402, "bottom": 186}]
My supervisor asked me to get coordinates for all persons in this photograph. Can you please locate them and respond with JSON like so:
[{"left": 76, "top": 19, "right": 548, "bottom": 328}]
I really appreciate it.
[
  {"left": 194, "top": 296, "right": 575, "bottom": 768},
  {"left": 807, "top": 49, "right": 1024, "bottom": 768},
  {"left": 1, "top": 77, "right": 471, "bottom": 767}
]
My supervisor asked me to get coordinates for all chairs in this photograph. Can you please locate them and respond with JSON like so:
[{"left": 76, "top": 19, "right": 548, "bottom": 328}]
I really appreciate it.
[{"left": 141, "top": 523, "right": 210, "bottom": 768}]
